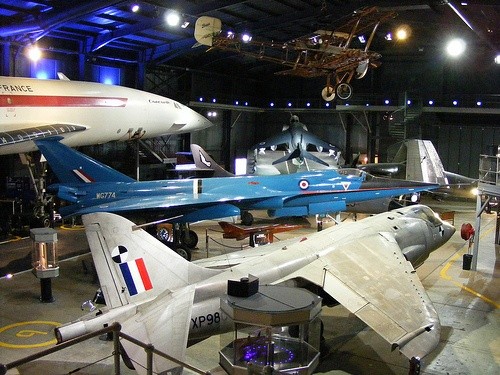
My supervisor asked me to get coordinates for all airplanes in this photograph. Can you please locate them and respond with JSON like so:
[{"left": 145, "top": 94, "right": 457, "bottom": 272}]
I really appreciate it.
[{"left": 0, "top": 2, "right": 499, "bottom": 375}]
